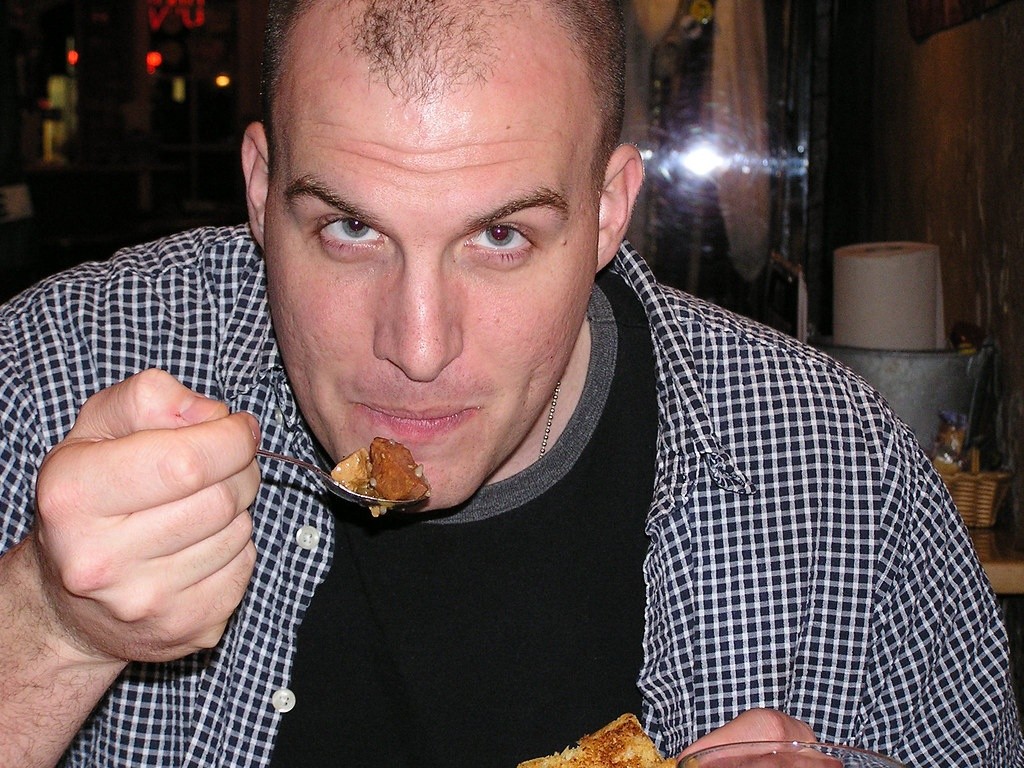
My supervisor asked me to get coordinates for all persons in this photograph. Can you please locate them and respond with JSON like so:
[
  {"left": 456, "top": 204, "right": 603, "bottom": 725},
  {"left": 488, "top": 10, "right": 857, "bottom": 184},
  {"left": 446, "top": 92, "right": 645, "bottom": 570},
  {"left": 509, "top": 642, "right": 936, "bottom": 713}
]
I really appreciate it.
[{"left": 0, "top": 0, "right": 1021, "bottom": 768}]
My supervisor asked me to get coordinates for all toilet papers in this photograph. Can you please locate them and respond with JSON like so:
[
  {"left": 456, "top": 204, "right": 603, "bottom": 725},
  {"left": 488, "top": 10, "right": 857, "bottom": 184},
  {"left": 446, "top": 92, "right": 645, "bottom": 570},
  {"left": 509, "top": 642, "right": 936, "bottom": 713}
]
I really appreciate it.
[{"left": 830, "top": 239, "right": 948, "bottom": 352}]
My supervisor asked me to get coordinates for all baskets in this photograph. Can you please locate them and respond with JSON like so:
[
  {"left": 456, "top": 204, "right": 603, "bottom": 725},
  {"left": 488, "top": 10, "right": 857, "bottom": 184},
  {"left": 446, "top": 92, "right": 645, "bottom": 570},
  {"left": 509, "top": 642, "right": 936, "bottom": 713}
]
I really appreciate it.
[
  {"left": 967, "top": 528, "right": 1001, "bottom": 562},
  {"left": 937, "top": 449, "right": 1014, "bottom": 526}
]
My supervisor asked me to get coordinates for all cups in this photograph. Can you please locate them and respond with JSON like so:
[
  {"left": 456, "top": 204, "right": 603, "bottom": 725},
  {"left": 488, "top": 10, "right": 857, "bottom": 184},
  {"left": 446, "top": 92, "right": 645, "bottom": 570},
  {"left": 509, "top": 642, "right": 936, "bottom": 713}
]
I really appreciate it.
[{"left": 678, "top": 741, "right": 909, "bottom": 768}]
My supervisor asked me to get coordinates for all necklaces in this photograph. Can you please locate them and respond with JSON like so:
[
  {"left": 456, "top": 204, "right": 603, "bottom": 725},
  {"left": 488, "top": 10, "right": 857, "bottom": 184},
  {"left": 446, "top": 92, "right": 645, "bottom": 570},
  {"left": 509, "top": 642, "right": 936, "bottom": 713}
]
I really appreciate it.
[{"left": 538, "top": 382, "right": 561, "bottom": 460}]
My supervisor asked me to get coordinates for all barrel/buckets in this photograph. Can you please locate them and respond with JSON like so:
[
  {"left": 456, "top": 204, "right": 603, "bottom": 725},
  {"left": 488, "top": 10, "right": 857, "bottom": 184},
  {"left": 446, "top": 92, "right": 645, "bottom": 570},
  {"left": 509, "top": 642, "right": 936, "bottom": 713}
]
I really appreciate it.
[{"left": 808, "top": 335, "right": 981, "bottom": 466}]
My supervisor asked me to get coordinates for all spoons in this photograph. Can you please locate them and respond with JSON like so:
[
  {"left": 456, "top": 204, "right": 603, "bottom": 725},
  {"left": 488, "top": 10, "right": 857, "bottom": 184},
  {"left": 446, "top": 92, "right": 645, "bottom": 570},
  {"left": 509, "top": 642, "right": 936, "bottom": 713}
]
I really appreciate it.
[{"left": 255, "top": 449, "right": 429, "bottom": 510}]
